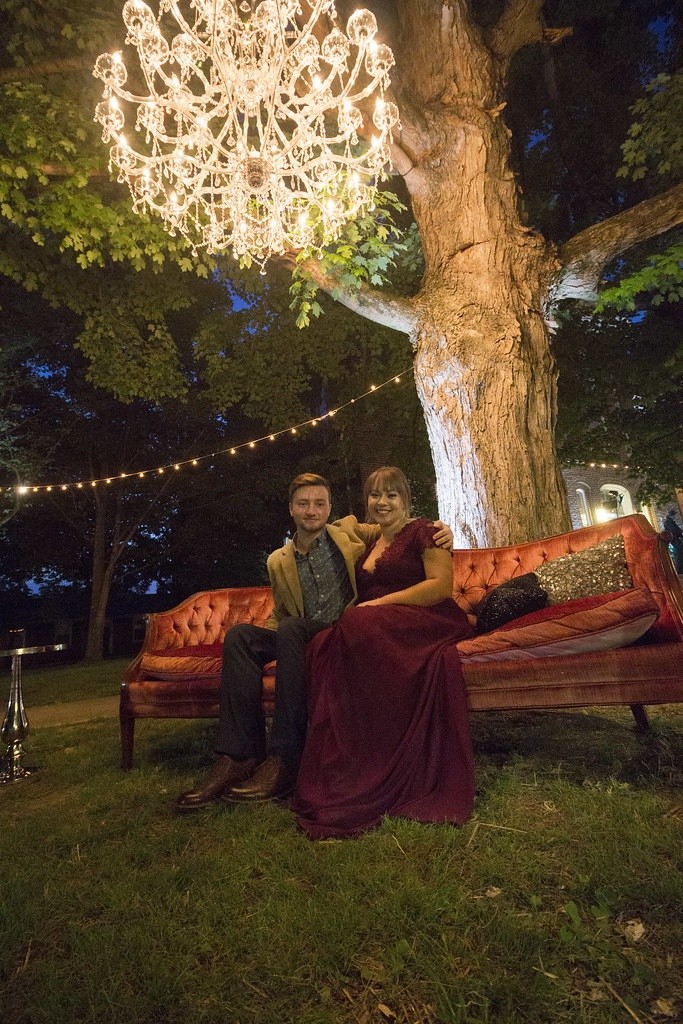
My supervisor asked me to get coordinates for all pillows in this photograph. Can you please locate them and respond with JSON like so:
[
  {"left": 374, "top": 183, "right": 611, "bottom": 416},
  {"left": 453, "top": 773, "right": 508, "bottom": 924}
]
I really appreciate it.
[
  {"left": 533, "top": 534, "right": 633, "bottom": 607},
  {"left": 476, "top": 572, "right": 550, "bottom": 639}
]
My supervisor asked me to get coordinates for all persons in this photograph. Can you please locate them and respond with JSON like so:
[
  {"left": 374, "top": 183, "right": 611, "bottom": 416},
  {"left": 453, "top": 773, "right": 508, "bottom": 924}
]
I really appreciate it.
[{"left": 172, "top": 466, "right": 476, "bottom": 842}]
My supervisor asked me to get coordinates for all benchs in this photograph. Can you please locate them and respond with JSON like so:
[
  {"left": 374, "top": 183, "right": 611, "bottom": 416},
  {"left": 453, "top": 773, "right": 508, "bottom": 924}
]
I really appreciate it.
[{"left": 119, "top": 515, "right": 683, "bottom": 774}]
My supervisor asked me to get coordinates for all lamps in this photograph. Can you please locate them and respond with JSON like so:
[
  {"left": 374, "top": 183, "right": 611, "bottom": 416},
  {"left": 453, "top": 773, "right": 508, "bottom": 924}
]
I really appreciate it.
[{"left": 92, "top": 0, "right": 403, "bottom": 275}]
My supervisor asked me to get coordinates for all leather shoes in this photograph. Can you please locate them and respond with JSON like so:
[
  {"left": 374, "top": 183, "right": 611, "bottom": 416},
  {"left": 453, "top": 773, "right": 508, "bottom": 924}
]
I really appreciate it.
[
  {"left": 221, "top": 755, "right": 296, "bottom": 804},
  {"left": 175, "top": 755, "right": 254, "bottom": 811}
]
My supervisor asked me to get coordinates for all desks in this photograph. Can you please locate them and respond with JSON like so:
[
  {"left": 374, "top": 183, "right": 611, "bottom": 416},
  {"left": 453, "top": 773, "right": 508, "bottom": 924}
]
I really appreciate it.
[{"left": 0, "top": 644, "right": 66, "bottom": 787}]
